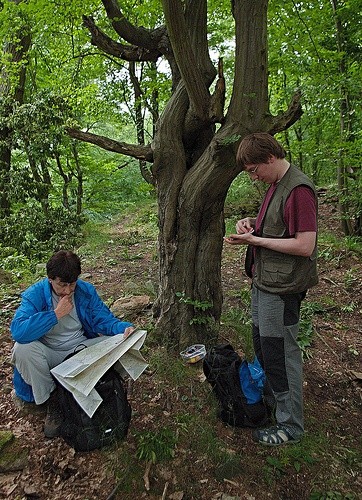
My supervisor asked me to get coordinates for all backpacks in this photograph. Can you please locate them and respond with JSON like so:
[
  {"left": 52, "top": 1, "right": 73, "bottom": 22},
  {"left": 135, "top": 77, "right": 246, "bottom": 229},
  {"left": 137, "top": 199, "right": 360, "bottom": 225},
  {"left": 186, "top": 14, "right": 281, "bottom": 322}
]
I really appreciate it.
[
  {"left": 57, "top": 344, "right": 131, "bottom": 452},
  {"left": 203, "top": 344, "right": 272, "bottom": 429}
]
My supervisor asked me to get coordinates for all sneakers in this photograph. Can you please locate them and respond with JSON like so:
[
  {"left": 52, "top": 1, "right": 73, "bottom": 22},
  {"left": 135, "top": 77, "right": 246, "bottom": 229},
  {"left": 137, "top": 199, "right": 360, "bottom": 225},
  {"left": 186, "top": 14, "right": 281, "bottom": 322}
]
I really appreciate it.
[
  {"left": 252, "top": 425, "right": 300, "bottom": 446},
  {"left": 44, "top": 393, "right": 62, "bottom": 438}
]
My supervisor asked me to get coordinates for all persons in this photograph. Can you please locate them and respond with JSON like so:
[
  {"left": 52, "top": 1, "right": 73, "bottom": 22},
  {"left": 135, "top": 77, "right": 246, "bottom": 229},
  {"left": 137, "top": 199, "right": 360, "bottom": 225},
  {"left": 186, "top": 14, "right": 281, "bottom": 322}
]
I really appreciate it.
[
  {"left": 222, "top": 132, "right": 318, "bottom": 447},
  {"left": 10, "top": 250, "right": 135, "bottom": 438}
]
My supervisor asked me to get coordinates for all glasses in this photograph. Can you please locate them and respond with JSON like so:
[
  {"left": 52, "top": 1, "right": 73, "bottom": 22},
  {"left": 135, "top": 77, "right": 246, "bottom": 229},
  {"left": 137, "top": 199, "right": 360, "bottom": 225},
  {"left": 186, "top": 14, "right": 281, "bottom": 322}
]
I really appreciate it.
[{"left": 247, "top": 163, "right": 260, "bottom": 177}]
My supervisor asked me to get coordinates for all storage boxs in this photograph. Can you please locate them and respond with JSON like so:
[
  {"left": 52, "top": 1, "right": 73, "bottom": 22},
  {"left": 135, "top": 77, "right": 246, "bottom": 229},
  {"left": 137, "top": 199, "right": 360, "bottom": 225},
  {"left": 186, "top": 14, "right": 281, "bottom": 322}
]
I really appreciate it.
[{"left": 180, "top": 344, "right": 207, "bottom": 363}]
